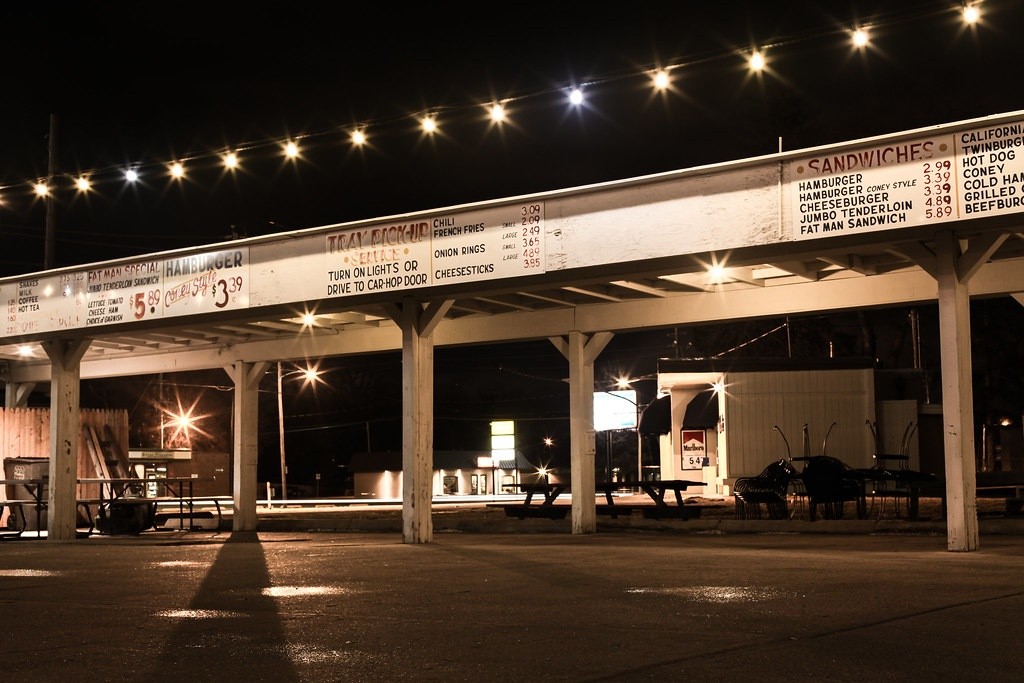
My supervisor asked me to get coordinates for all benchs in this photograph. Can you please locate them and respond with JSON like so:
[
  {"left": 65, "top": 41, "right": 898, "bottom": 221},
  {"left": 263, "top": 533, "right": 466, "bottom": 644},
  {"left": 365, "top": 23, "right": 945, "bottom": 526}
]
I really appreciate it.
[
  {"left": 0, "top": 496, "right": 235, "bottom": 541},
  {"left": 502, "top": 504, "right": 707, "bottom": 521}
]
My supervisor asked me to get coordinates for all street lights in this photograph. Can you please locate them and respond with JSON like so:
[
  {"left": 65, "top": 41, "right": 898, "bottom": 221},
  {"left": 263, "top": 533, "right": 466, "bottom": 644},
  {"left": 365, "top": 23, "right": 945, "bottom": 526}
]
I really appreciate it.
[
  {"left": 277, "top": 361, "right": 316, "bottom": 497},
  {"left": 983, "top": 420, "right": 1010, "bottom": 472}
]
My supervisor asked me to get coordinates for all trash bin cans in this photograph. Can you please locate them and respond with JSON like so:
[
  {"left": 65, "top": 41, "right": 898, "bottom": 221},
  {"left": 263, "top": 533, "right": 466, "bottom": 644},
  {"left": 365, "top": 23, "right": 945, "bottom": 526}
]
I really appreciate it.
[{"left": 3, "top": 456, "right": 49, "bottom": 532}]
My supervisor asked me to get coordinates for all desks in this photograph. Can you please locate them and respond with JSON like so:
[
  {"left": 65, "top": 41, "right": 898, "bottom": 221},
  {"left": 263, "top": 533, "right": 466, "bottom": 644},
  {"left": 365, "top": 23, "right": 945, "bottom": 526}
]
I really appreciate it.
[
  {"left": 502, "top": 480, "right": 708, "bottom": 521},
  {"left": 0, "top": 476, "right": 216, "bottom": 538}
]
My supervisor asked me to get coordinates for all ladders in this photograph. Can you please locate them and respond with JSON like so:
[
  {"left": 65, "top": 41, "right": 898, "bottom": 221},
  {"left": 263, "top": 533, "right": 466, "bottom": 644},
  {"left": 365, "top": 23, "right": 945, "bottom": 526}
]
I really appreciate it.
[{"left": 80, "top": 423, "right": 147, "bottom": 500}]
